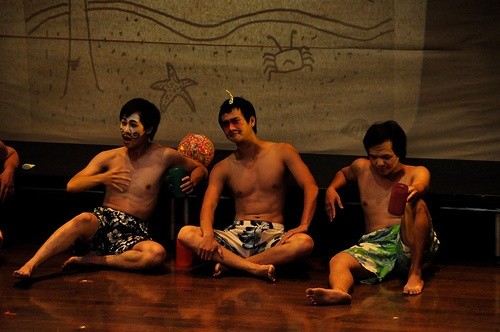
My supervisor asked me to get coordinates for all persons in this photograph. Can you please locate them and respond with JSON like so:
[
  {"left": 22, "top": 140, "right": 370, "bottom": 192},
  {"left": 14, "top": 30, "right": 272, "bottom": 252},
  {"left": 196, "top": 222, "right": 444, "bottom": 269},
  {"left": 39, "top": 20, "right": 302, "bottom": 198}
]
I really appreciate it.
[
  {"left": 13, "top": 97, "right": 208, "bottom": 279},
  {"left": 306, "top": 120, "right": 440, "bottom": 305},
  {"left": 177, "top": 97, "right": 319, "bottom": 282},
  {"left": 0, "top": 140, "right": 19, "bottom": 243}
]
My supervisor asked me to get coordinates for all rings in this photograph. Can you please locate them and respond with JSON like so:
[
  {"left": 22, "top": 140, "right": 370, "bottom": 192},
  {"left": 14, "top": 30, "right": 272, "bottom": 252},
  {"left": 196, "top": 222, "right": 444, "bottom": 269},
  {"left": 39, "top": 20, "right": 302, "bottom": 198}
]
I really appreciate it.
[
  {"left": 408, "top": 188, "right": 412, "bottom": 193},
  {"left": 326, "top": 206, "right": 331, "bottom": 212}
]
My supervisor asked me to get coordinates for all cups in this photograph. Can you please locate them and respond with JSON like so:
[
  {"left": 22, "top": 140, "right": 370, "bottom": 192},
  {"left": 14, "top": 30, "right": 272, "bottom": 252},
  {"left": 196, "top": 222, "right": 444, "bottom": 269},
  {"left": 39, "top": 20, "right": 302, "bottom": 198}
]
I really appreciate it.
[
  {"left": 166, "top": 165, "right": 186, "bottom": 199},
  {"left": 388, "top": 183, "right": 408, "bottom": 216}
]
